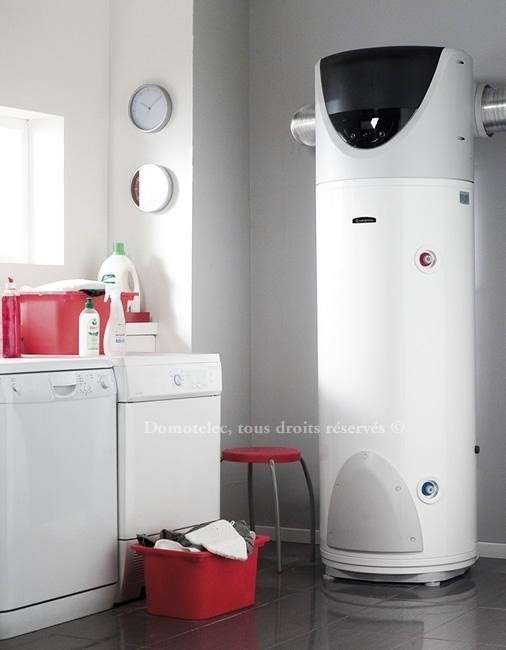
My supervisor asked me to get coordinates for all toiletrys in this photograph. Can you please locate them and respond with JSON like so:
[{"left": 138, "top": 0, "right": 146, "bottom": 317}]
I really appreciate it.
[
  {"left": 2, "top": 276, "right": 22, "bottom": 357},
  {"left": 77, "top": 297, "right": 101, "bottom": 359}
]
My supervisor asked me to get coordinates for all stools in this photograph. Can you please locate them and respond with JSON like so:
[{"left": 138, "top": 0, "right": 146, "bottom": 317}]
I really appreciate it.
[{"left": 219, "top": 445, "right": 322, "bottom": 577}]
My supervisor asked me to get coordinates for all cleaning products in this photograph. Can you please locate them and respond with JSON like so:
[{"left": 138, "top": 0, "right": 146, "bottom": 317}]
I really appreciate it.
[
  {"left": 97, "top": 242, "right": 141, "bottom": 311},
  {"left": 103, "top": 283, "right": 127, "bottom": 357}
]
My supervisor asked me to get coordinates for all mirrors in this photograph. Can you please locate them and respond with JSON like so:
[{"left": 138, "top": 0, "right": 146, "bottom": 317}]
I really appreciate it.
[{"left": 129, "top": 162, "right": 176, "bottom": 215}]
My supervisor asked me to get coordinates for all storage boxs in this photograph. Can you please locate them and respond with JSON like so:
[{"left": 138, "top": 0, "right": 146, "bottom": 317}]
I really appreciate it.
[
  {"left": 16, "top": 277, "right": 140, "bottom": 359},
  {"left": 122, "top": 527, "right": 275, "bottom": 621}
]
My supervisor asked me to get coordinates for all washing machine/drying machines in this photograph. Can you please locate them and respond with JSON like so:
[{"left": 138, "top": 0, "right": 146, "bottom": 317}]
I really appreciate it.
[
  {"left": 117, "top": 350, "right": 224, "bottom": 600},
  {"left": 2, "top": 352, "right": 118, "bottom": 643}
]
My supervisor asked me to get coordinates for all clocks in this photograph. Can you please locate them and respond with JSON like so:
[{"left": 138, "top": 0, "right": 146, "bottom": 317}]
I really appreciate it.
[{"left": 128, "top": 80, "right": 174, "bottom": 134}]
